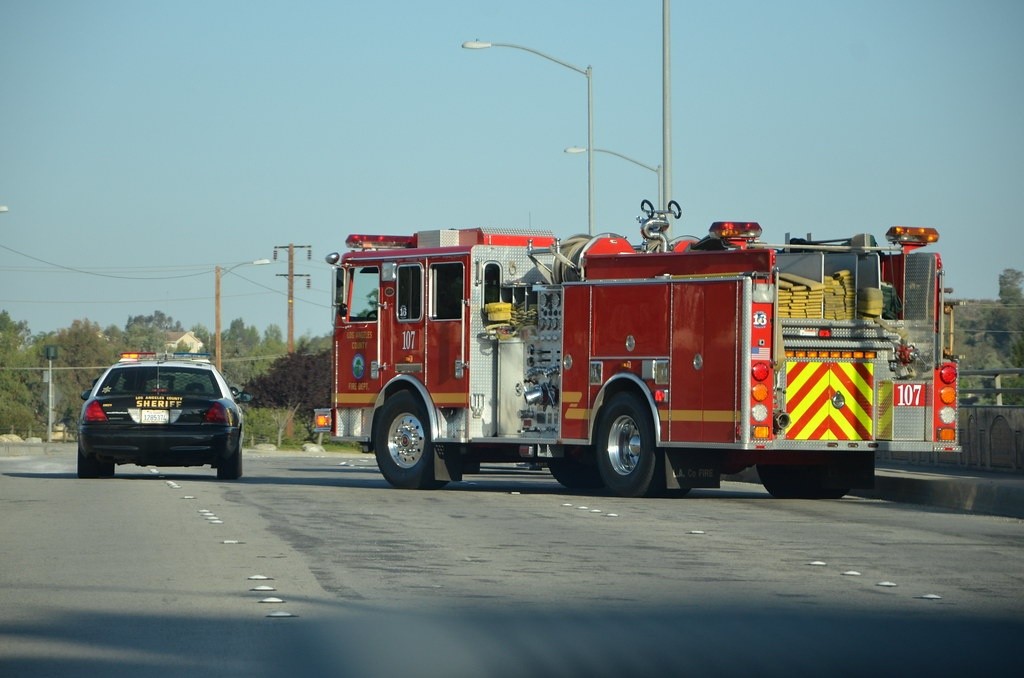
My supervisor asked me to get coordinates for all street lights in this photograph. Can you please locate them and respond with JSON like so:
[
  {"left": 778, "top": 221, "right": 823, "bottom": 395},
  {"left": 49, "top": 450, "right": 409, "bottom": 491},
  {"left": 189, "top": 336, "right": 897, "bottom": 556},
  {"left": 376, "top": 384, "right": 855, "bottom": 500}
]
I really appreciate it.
[
  {"left": 213, "top": 258, "right": 272, "bottom": 375},
  {"left": 564, "top": 142, "right": 662, "bottom": 221},
  {"left": 461, "top": 36, "right": 596, "bottom": 237}
]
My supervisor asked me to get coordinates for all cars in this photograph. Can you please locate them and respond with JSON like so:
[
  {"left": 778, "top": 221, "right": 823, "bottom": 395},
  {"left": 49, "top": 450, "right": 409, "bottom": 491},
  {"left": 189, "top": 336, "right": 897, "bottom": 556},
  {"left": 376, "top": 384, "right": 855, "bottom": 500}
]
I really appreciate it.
[{"left": 77, "top": 354, "right": 246, "bottom": 481}]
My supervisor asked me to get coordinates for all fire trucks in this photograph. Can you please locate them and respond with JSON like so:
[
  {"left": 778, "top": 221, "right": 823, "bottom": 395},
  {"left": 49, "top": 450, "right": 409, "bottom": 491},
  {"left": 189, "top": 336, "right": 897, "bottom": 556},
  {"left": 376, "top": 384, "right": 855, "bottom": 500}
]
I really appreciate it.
[{"left": 311, "top": 208, "right": 961, "bottom": 505}]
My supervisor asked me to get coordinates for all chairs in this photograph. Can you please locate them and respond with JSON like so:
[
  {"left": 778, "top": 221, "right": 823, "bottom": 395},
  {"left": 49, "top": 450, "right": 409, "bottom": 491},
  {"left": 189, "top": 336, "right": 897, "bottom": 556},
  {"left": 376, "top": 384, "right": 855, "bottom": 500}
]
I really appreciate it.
[
  {"left": 186, "top": 383, "right": 205, "bottom": 394},
  {"left": 123, "top": 380, "right": 147, "bottom": 394}
]
